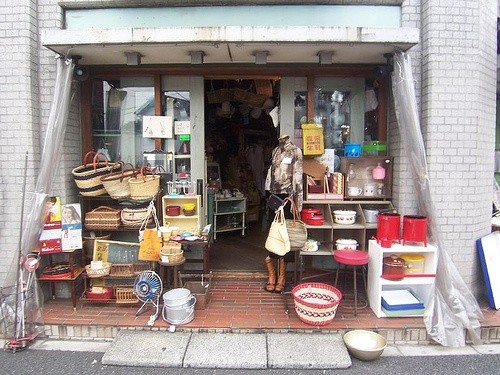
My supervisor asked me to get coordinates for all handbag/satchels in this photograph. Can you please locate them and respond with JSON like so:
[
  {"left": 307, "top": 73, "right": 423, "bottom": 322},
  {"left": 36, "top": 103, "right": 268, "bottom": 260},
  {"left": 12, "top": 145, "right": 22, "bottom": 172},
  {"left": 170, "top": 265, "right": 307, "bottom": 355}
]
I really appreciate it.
[
  {"left": 136, "top": 205, "right": 164, "bottom": 262},
  {"left": 268, "top": 193, "right": 286, "bottom": 213},
  {"left": 95, "top": 138, "right": 112, "bottom": 162},
  {"left": 265, "top": 164, "right": 273, "bottom": 191},
  {"left": 265, "top": 206, "right": 291, "bottom": 256},
  {"left": 281, "top": 197, "right": 308, "bottom": 251}
]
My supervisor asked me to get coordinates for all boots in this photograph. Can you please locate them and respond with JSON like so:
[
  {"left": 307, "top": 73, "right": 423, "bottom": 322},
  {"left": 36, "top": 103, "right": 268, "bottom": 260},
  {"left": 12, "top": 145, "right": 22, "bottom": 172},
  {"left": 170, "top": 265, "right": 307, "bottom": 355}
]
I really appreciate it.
[{"left": 264, "top": 256, "right": 287, "bottom": 293}]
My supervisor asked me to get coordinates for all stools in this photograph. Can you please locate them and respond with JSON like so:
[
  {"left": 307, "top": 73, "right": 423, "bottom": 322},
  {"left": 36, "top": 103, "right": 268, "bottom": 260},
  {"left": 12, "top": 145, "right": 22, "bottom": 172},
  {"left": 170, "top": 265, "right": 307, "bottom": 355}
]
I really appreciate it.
[{"left": 334, "top": 249, "right": 368, "bottom": 318}]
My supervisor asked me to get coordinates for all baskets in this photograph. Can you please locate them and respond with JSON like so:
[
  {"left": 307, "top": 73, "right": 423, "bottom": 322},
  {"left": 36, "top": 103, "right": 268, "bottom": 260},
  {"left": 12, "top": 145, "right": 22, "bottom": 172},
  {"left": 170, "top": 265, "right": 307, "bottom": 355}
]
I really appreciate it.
[
  {"left": 99, "top": 161, "right": 138, "bottom": 199},
  {"left": 206, "top": 79, "right": 237, "bottom": 103},
  {"left": 235, "top": 88, "right": 266, "bottom": 108},
  {"left": 112, "top": 263, "right": 135, "bottom": 278},
  {"left": 159, "top": 249, "right": 183, "bottom": 263},
  {"left": 84, "top": 205, "right": 121, "bottom": 230},
  {"left": 176, "top": 271, "right": 214, "bottom": 310},
  {"left": 254, "top": 80, "right": 273, "bottom": 97},
  {"left": 85, "top": 262, "right": 111, "bottom": 278},
  {"left": 120, "top": 207, "right": 154, "bottom": 227},
  {"left": 82, "top": 230, "right": 112, "bottom": 260},
  {"left": 85, "top": 287, "right": 113, "bottom": 303},
  {"left": 72, "top": 150, "right": 121, "bottom": 196},
  {"left": 128, "top": 167, "right": 160, "bottom": 201},
  {"left": 116, "top": 287, "right": 139, "bottom": 304},
  {"left": 292, "top": 283, "right": 342, "bottom": 325}
]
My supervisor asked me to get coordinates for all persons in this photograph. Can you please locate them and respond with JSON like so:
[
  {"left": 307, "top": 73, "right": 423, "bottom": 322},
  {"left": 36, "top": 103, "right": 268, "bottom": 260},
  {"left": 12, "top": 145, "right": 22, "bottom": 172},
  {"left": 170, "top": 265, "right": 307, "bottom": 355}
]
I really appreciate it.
[
  {"left": 62, "top": 204, "right": 81, "bottom": 223},
  {"left": 43, "top": 197, "right": 57, "bottom": 223},
  {"left": 262, "top": 134, "right": 303, "bottom": 292}
]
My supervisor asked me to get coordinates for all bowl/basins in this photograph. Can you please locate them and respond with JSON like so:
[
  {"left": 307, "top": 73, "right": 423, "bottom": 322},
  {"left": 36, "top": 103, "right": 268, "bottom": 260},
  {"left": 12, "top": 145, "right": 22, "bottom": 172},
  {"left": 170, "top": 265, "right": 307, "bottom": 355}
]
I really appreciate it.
[
  {"left": 343, "top": 330, "right": 388, "bottom": 361},
  {"left": 299, "top": 208, "right": 380, "bottom": 253},
  {"left": 347, "top": 162, "right": 387, "bottom": 196},
  {"left": 382, "top": 254, "right": 426, "bottom": 280}
]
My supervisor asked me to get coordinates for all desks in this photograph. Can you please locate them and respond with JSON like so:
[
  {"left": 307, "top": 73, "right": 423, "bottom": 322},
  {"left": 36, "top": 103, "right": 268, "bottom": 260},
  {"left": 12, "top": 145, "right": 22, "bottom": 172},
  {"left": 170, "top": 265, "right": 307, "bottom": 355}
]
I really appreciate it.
[{"left": 172, "top": 234, "right": 212, "bottom": 286}]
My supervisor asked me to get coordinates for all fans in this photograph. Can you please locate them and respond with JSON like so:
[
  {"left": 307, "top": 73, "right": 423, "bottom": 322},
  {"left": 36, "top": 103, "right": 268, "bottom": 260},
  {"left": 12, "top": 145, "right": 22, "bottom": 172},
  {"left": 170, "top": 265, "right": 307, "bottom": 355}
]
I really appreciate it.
[{"left": 132, "top": 270, "right": 163, "bottom": 327}]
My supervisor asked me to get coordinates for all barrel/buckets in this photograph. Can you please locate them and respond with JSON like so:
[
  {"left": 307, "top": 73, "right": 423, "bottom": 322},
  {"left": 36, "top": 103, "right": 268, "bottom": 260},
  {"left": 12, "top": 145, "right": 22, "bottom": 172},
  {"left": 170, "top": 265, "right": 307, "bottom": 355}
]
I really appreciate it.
[{"left": 162, "top": 288, "right": 196, "bottom": 326}]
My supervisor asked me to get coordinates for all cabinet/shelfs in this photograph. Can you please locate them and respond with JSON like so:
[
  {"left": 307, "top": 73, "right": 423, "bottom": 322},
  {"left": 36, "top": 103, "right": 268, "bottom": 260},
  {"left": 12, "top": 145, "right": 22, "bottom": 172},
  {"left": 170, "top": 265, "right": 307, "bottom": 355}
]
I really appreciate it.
[
  {"left": 295, "top": 199, "right": 395, "bottom": 277},
  {"left": 212, "top": 197, "right": 248, "bottom": 243},
  {"left": 76, "top": 189, "right": 165, "bottom": 309},
  {"left": 162, "top": 195, "right": 203, "bottom": 236},
  {"left": 368, "top": 240, "right": 439, "bottom": 318},
  {"left": 342, "top": 156, "right": 394, "bottom": 201},
  {"left": 31, "top": 241, "right": 85, "bottom": 311}
]
